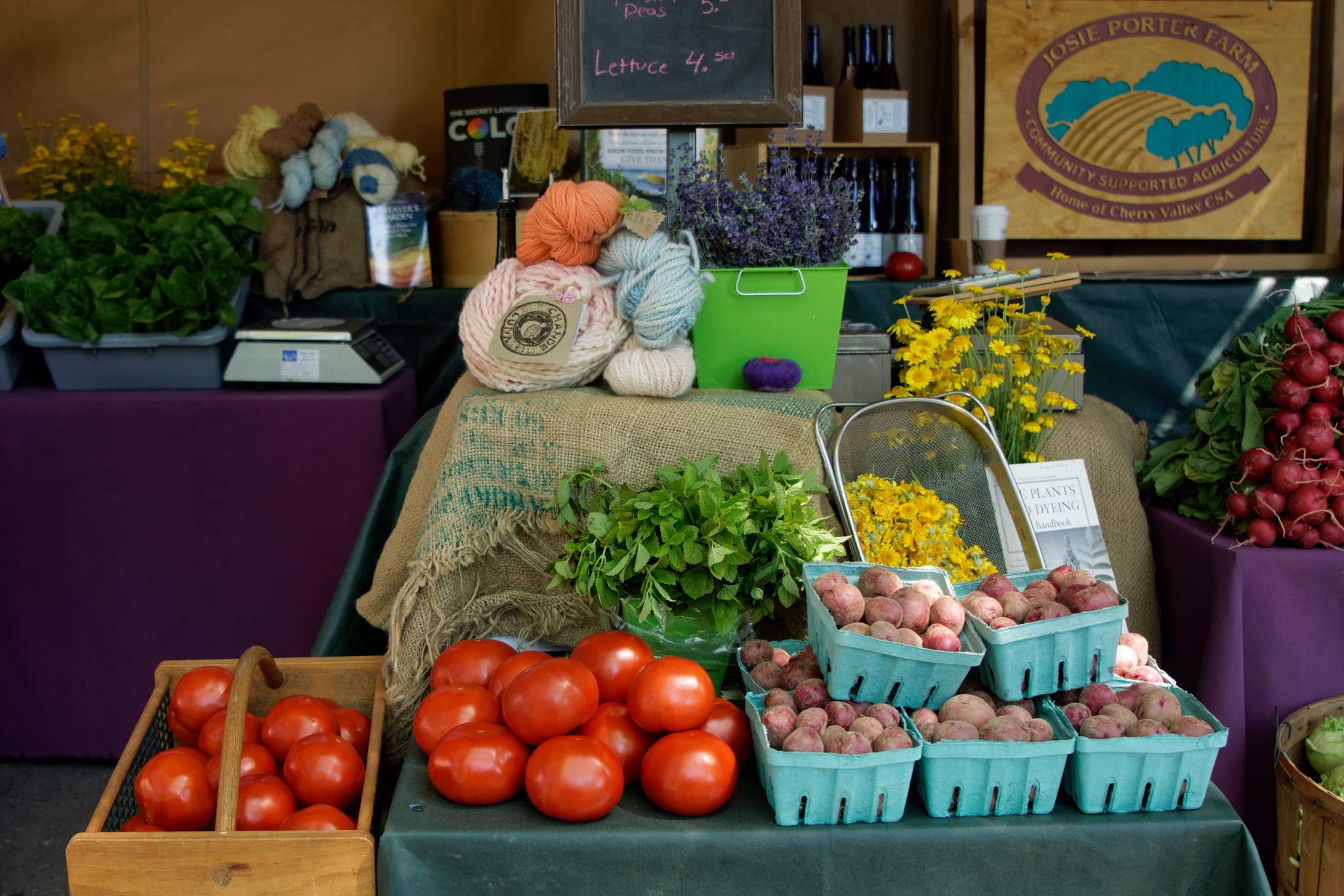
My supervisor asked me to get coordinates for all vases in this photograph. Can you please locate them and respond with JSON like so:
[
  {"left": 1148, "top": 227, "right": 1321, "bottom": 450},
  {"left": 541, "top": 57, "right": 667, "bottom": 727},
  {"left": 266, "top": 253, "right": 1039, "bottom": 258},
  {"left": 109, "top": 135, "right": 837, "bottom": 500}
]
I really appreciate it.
[{"left": 677, "top": 259, "right": 852, "bottom": 388}]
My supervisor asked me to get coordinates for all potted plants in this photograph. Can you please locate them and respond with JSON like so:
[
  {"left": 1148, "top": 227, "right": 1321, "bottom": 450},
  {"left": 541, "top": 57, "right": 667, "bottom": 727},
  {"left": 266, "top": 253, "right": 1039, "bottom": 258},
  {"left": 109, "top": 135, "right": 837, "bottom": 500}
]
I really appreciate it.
[
  {"left": 545, "top": 447, "right": 854, "bottom": 694},
  {"left": 0, "top": 197, "right": 64, "bottom": 393},
  {"left": 2, "top": 177, "right": 272, "bottom": 392}
]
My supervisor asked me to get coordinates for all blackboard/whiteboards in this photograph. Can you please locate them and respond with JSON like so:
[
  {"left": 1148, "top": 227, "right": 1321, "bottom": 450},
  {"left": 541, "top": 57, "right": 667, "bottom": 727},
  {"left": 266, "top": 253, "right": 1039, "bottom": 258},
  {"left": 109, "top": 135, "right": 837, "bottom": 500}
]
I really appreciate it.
[{"left": 556, "top": 0, "right": 802, "bottom": 127}]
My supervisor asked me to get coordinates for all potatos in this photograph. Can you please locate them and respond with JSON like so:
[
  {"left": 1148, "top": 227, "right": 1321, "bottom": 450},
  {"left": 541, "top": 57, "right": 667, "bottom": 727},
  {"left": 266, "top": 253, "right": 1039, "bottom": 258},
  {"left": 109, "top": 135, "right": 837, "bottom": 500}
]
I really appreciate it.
[{"left": 742, "top": 563, "right": 1213, "bottom": 823}]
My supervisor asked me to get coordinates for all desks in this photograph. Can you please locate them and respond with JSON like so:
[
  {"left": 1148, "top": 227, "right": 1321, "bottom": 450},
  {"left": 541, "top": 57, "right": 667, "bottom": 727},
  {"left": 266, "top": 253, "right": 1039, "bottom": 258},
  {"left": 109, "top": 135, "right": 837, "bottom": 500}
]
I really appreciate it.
[
  {"left": 0, "top": 352, "right": 414, "bottom": 761},
  {"left": 844, "top": 269, "right": 1344, "bottom": 458},
  {"left": 1143, "top": 497, "right": 1344, "bottom": 896},
  {"left": 374, "top": 671, "right": 1279, "bottom": 896},
  {"left": 351, "top": 364, "right": 848, "bottom": 649}
]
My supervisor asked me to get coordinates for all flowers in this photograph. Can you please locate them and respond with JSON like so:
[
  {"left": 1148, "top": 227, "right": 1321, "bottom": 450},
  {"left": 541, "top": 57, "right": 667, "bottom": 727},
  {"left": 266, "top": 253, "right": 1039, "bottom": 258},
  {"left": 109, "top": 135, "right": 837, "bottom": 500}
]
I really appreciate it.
[
  {"left": 2, "top": 101, "right": 218, "bottom": 203},
  {"left": 841, "top": 250, "right": 1097, "bottom": 585},
  {"left": 658, "top": 105, "right": 865, "bottom": 271}
]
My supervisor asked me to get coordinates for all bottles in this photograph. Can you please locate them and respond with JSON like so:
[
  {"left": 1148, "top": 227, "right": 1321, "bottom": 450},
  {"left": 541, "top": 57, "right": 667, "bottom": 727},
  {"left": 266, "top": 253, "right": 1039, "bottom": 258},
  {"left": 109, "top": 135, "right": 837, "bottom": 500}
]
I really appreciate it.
[
  {"left": 838, "top": 20, "right": 897, "bottom": 89},
  {"left": 795, "top": 154, "right": 921, "bottom": 274},
  {"left": 806, "top": 23, "right": 821, "bottom": 87},
  {"left": 492, "top": 197, "right": 516, "bottom": 265}
]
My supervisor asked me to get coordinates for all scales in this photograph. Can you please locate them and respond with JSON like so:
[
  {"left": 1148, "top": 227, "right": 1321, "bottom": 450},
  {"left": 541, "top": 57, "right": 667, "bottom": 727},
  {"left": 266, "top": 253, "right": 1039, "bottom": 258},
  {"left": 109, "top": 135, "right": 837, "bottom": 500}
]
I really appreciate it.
[{"left": 221, "top": 319, "right": 406, "bottom": 387}]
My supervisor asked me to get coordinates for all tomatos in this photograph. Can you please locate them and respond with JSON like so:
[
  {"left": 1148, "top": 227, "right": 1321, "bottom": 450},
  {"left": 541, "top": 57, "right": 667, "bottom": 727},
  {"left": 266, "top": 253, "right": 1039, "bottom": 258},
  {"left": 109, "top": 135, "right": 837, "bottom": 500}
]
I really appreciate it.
[
  {"left": 883, "top": 251, "right": 923, "bottom": 280},
  {"left": 117, "top": 630, "right": 745, "bottom": 833}
]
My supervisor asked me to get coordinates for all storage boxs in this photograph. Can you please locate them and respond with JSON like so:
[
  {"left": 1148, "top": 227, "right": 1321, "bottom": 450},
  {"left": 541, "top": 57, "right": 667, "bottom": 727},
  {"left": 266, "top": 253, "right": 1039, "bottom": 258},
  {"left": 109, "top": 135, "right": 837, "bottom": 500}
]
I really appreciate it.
[
  {"left": 1276, "top": 692, "right": 1344, "bottom": 896},
  {"left": 736, "top": 559, "right": 1229, "bottom": 830},
  {"left": 65, "top": 642, "right": 389, "bottom": 896}
]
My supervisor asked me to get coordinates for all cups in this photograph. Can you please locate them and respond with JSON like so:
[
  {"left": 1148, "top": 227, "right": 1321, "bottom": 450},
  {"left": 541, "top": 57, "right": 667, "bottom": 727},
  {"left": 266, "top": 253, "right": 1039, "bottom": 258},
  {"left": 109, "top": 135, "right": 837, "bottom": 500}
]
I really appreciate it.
[{"left": 972, "top": 201, "right": 1007, "bottom": 269}]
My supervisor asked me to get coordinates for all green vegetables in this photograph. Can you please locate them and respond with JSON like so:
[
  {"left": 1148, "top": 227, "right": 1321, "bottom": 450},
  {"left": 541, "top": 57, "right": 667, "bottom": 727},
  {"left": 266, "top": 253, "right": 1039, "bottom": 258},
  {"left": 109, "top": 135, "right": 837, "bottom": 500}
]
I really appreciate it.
[
  {"left": 541, "top": 448, "right": 854, "bottom": 634},
  {"left": 0, "top": 178, "right": 267, "bottom": 343}
]
